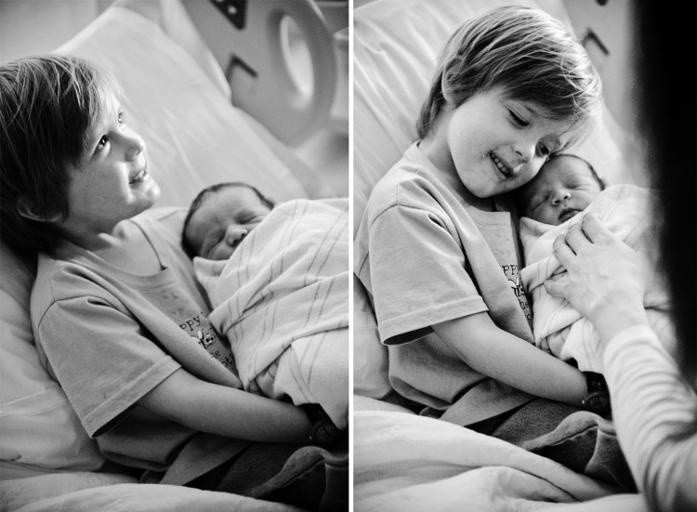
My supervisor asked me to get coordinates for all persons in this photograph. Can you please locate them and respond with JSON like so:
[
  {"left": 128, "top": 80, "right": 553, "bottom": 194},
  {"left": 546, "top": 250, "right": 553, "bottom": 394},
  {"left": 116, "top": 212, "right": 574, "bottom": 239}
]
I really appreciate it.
[
  {"left": 353, "top": 2, "right": 634, "bottom": 494},
  {"left": 180, "top": 182, "right": 348, "bottom": 414},
  {"left": 523, "top": 152, "right": 657, "bottom": 360},
  {"left": 543, "top": 215, "right": 696, "bottom": 512}
]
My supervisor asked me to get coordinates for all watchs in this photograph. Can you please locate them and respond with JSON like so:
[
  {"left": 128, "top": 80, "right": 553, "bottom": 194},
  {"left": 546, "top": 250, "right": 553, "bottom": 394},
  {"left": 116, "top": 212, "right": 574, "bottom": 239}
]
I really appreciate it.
[
  {"left": 582, "top": 371, "right": 610, "bottom": 417},
  {"left": 304, "top": 406, "right": 336, "bottom": 450}
]
